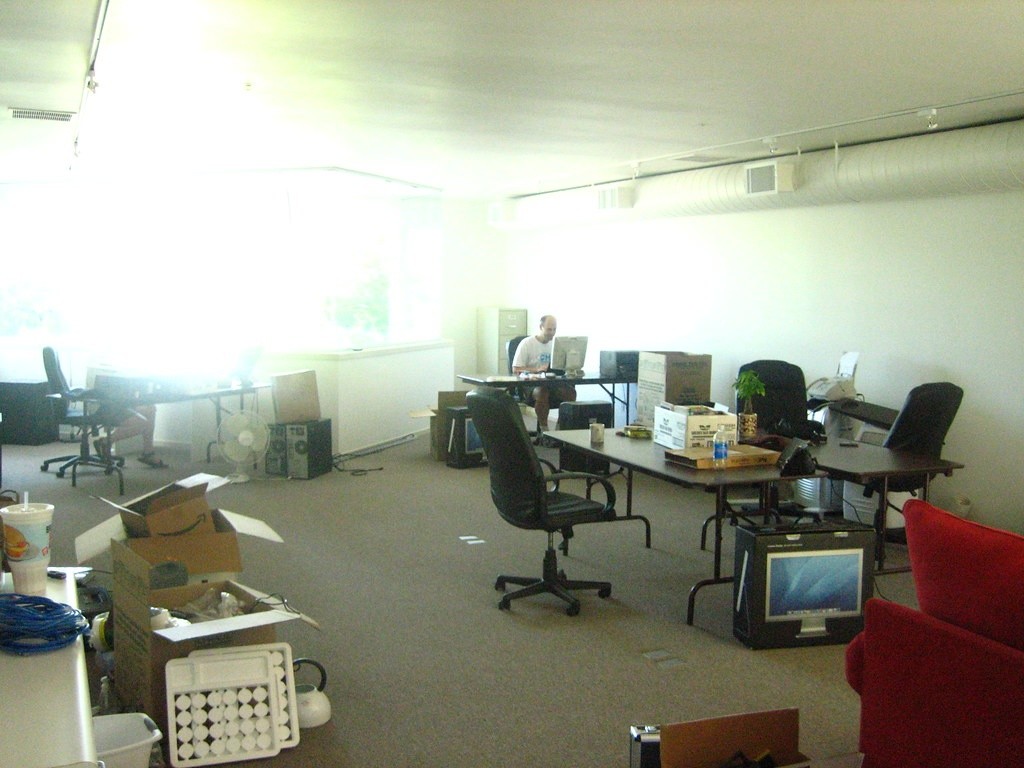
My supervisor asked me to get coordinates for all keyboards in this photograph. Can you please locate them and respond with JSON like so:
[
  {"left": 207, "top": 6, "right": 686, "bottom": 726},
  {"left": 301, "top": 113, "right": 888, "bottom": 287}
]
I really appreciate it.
[{"left": 547, "top": 368, "right": 565, "bottom": 376}]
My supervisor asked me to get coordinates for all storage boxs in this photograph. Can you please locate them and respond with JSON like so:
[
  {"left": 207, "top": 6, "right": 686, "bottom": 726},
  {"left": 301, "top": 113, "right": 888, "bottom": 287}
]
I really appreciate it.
[
  {"left": 271, "top": 367, "right": 321, "bottom": 425},
  {"left": 661, "top": 443, "right": 783, "bottom": 472},
  {"left": 659, "top": 706, "right": 815, "bottom": 768},
  {"left": 407, "top": 388, "right": 501, "bottom": 468},
  {"left": 731, "top": 519, "right": 882, "bottom": 650},
  {"left": 651, "top": 401, "right": 740, "bottom": 447},
  {"left": 636, "top": 352, "right": 714, "bottom": 427},
  {"left": 81, "top": 365, "right": 156, "bottom": 455},
  {"left": 75, "top": 471, "right": 321, "bottom": 768}
]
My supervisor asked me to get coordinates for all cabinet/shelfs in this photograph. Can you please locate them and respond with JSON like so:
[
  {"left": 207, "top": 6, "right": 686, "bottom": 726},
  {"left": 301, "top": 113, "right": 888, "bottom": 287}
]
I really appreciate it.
[{"left": 475, "top": 306, "right": 528, "bottom": 377}]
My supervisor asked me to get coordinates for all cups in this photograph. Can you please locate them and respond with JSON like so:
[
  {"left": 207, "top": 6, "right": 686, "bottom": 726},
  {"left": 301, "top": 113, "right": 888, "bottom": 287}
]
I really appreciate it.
[
  {"left": 590, "top": 424, "right": 605, "bottom": 443},
  {"left": 0, "top": 503, "right": 54, "bottom": 597}
]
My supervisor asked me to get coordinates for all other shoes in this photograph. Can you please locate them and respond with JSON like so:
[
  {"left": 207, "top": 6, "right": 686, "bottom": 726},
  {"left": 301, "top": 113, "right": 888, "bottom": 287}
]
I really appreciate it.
[{"left": 537, "top": 436, "right": 562, "bottom": 448}]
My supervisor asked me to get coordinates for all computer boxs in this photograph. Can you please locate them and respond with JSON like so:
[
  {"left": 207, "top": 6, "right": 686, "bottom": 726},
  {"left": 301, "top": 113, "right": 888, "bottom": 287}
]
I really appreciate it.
[{"left": 265, "top": 418, "right": 333, "bottom": 480}]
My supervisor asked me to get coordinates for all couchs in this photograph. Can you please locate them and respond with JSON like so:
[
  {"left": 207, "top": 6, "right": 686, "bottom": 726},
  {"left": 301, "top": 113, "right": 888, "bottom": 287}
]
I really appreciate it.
[{"left": 846, "top": 500, "right": 1024, "bottom": 768}]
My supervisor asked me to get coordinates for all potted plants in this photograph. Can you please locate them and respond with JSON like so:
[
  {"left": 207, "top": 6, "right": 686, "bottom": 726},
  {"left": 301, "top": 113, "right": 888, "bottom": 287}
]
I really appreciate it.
[{"left": 734, "top": 369, "right": 766, "bottom": 438}]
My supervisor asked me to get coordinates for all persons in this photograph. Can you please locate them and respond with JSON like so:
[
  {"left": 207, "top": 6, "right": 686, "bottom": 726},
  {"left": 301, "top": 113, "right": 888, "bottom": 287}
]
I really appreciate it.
[
  {"left": 86, "top": 371, "right": 168, "bottom": 466},
  {"left": 511, "top": 315, "right": 575, "bottom": 448}
]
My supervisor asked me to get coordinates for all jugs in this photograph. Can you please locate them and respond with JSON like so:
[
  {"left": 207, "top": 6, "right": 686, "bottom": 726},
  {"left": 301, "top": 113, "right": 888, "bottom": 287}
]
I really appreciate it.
[{"left": 293, "top": 658, "right": 331, "bottom": 729}]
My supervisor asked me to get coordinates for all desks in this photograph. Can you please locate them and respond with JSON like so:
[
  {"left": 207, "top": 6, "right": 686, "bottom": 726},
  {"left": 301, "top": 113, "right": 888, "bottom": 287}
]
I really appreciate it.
[
  {"left": 456, "top": 371, "right": 636, "bottom": 430},
  {"left": 0, "top": 563, "right": 97, "bottom": 768},
  {"left": 700, "top": 415, "right": 967, "bottom": 574},
  {"left": 45, "top": 381, "right": 268, "bottom": 495},
  {"left": 542, "top": 422, "right": 832, "bottom": 625}
]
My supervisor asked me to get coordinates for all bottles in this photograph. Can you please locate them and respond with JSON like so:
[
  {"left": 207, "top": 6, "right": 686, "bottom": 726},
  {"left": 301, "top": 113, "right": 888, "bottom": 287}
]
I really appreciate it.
[{"left": 712, "top": 425, "right": 727, "bottom": 471}]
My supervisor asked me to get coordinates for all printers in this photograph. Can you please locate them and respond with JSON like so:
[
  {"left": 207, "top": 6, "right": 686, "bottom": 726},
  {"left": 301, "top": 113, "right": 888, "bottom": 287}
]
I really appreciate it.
[
  {"left": 599, "top": 350, "right": 640, "bottom": 381},
  {"left": 794, "top": 363, "right": 866, "bottom": 521}
]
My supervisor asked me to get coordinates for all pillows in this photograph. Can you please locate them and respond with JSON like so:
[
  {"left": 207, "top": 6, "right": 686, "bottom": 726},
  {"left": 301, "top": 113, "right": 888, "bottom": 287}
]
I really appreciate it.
[{"left": 903, "top": 500, "right": 1024, "bottom": 651}]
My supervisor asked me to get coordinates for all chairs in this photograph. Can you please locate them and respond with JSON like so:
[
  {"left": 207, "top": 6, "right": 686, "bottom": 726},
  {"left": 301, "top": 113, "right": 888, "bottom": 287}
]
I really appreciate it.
[
  {"left": 860, "top": 383, "right": 964, "bottom": 566},
  {"left": 505, "top": 336, "right": 578, "bottom": 446},
  {"left": 731, "top": 360, "right": 829, "bottom": 532},
  {"left": 40, "top": 346, "right": 126, "bottom": 478},
  {"left": 466, "top": 387, "right": 620, "bottom": 619}
]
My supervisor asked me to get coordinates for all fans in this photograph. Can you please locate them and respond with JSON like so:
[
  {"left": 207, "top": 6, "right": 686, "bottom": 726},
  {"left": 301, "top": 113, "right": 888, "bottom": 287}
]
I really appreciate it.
[{"left": 216, "top": 410, "right": 271, "bottom": 486}]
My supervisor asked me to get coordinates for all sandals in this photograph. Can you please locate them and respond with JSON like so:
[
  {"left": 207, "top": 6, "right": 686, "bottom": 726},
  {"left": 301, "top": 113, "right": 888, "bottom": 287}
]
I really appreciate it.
[
  {"left": 94, "top": 437, "right": 107, "bottom": 459},
  {"left": 137, "top": 451, "right": 169, "bottom": 468}
]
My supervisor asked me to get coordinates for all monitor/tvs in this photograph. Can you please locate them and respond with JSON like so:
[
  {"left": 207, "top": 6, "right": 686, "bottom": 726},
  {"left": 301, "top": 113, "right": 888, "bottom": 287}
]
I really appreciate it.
[
  {"left": 551, "top": 336, "right": 588, "bottom": 380},
  {"left": 464, "top": 418, "right": 488, "bottom": 462},
  {"left": 764, "top": 549, "right": 863, "bottom": 638}
]
